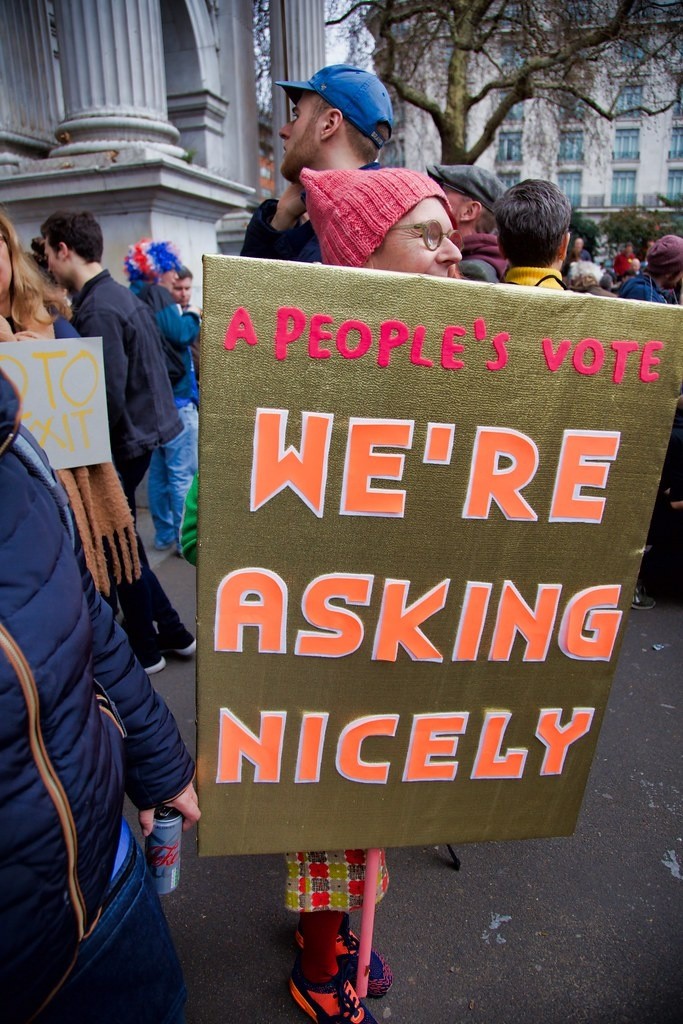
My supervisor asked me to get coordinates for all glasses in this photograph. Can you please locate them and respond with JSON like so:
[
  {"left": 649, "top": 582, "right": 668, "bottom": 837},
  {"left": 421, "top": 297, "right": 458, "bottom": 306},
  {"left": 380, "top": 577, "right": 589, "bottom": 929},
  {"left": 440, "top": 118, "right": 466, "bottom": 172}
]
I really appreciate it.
[{"left": 389, "top": 219, "right": 465, "bottom": 253}]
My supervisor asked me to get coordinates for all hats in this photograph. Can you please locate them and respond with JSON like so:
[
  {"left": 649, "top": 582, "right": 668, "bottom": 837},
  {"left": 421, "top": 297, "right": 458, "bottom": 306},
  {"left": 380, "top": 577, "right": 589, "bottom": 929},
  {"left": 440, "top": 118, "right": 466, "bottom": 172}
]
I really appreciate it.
[
  {"left": 123, "top": 238, "right": 181, "bottom": 285},
  {"left": 275, "top": 65, "right": 393, "bottom": 150},
  {"left": 426, "top": 163, "right": 508, "bottom": 215},
  {"left": 646, "top": 234, "right": 683, "bottom": 273},
  {"left": 298, "top": 166, "right": 458, "bottom": 268}
]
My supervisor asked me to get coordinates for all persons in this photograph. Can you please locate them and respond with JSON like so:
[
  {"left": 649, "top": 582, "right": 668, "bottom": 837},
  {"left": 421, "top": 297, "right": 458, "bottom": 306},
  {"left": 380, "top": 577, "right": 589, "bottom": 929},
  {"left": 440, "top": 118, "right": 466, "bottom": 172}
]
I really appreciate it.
[
  {"left": 237, "top": 63, "right": 393, "bottom": 264},
  {"left": 426, "top": 165, "right": 683, "bottom": 613},
  {"left": 282, "top": 165, "right": 462, "bottom": 1024},
  {"left": 0, "top": 208, "right": 204, "bottom": 1024}
]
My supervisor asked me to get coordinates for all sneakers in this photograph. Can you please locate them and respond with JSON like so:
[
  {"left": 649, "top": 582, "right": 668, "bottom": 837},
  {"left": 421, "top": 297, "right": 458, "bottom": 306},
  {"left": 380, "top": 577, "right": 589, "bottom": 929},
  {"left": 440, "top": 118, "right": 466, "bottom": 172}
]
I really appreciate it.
[
  {"left": 630, "top": 580, "right": 657, "bottom": 610},
  {"left": 290, "top": 950, "right": 380, "bottom": 1024},
  {"left": 295, "top": 913, "right": 393, "bottom": 997}
]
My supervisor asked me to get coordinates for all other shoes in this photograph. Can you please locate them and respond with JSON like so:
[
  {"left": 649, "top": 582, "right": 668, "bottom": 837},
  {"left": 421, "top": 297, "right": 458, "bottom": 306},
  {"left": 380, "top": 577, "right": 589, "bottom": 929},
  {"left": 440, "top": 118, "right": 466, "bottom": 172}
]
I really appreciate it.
[
  {"left": 158, "top": 625, "right": 197, "bottom": 655},
  {"left": 132, "top": 652, "right": 165, "bottom": 674}
]
